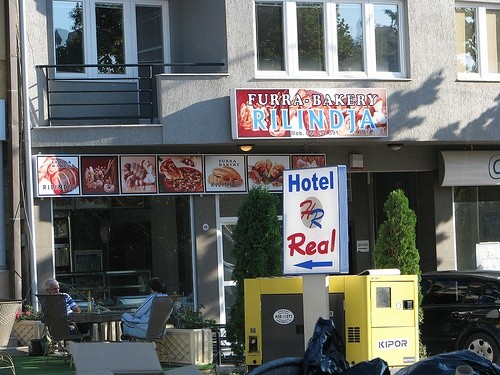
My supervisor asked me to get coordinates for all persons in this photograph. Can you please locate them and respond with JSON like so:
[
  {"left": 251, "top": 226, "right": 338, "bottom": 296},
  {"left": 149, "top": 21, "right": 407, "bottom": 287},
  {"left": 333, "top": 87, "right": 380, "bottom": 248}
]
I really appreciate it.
[
  {"left": 120, "top": 278, "right": 171, "bottom": 339},
  {"left": 45, "top": 280, "right": 93, "bottom": 342}
]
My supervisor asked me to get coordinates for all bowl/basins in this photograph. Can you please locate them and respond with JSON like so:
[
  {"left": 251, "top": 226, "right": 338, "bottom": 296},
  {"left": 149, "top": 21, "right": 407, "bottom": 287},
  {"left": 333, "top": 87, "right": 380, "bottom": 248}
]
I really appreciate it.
[{"left": 31, "top": 339, "right": 48, "bottom": 356}]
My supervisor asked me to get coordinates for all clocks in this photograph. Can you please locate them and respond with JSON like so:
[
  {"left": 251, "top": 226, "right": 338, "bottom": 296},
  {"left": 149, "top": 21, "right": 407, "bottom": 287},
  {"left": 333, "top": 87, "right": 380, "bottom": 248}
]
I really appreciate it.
[{"left": 99, "top": 220, "right": 111, "bottom": 245}]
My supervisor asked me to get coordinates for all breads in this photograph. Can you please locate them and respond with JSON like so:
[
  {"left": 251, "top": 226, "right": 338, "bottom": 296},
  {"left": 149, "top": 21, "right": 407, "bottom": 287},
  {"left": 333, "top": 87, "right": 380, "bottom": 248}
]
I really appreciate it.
[
  {"left": 38, "top": 158, "right": 78, "bottom": 194},
  {"left": 208, "top": 167, "right": 243, "bottom": 187},
  {"left": 123, "top": 160, "right": 156, "bottom": 187}
]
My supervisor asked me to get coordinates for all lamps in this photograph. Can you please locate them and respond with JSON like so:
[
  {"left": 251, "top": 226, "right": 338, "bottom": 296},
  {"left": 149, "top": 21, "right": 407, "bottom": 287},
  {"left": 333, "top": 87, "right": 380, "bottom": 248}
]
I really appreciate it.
[
  {"left": 387, "top": 144, "right": 404, "bottom": 152},
  {"left": 237, "top": 144, "right": 255, "bottom": 152}
]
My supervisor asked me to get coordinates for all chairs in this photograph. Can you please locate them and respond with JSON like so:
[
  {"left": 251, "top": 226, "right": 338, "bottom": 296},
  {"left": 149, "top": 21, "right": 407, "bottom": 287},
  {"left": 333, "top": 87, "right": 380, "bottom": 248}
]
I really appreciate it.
[
  {"left": 35, "top": 294, "right": 90, "bottom": 370},
  {"left": 121, "top": 295, "right": 180, "bottom": 367}
]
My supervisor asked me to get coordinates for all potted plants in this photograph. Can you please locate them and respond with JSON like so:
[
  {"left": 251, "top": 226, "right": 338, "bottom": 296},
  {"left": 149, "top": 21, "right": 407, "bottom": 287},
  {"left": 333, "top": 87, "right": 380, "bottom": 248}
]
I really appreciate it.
[{"left": 155, "top": 302, "right": 218, "bottom": 367}]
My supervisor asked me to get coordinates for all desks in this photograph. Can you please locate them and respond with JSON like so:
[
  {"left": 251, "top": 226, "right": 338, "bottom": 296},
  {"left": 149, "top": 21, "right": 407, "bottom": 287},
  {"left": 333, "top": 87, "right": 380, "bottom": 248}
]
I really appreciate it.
[{"left": 68, "top": 310, "right": 135, "bottom": 343}]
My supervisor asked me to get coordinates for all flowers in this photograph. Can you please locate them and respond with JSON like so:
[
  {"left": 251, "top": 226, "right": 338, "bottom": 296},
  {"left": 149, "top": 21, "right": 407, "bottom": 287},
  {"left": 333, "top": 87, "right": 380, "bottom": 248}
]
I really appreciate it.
[{"left": 15, "top": 303, "right": 43, "bottom": 322}]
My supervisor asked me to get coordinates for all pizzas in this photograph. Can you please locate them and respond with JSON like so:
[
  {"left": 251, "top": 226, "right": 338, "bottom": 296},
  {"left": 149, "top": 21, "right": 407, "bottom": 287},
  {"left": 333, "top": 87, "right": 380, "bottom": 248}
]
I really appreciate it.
[{"left": 159, "top": 159, "right": 204, "bottom": 192}]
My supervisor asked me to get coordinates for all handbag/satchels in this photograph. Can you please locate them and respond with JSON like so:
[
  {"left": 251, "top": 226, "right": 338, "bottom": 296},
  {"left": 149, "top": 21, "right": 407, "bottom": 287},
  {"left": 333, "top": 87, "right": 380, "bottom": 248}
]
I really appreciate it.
[{"left": 30, "top": 338, "right": 50, "bottom": 356}]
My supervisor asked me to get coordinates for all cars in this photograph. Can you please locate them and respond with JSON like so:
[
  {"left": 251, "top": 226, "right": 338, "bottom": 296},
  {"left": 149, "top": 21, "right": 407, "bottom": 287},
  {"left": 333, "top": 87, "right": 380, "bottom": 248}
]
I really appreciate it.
[{"left": 419, "top": 270, "right": 499, "bottom": 363}]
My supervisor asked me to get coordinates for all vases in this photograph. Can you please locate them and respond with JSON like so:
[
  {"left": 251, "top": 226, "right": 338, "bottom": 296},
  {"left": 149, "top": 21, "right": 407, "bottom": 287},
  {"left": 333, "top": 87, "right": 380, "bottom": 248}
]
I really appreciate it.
[
  {"left": 0, "top": 300, "right": 23, "bottom": 351},
  {"left": 11, "top": 319, "right": 45, "bottom": 352}
]
